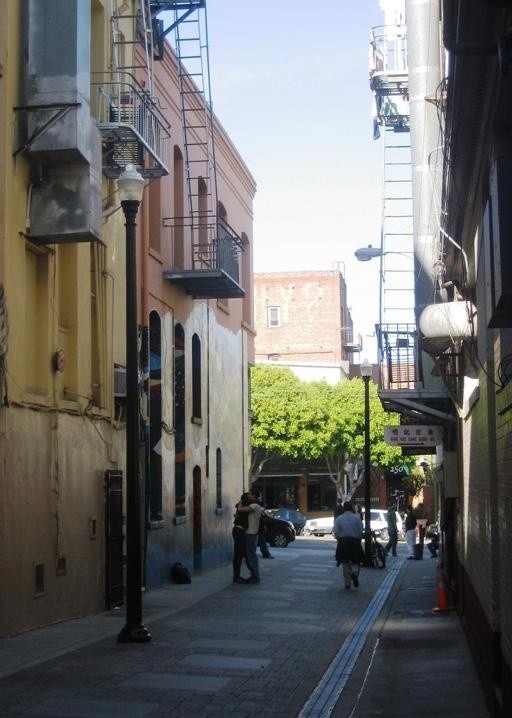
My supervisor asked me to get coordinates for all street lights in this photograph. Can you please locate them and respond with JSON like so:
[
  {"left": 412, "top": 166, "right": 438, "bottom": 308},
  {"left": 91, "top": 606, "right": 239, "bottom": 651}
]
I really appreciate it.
[
  {"left": 359, "top": 358, "right": 372, "bottom": 568},
  {"left": 114, "top": 161, "right": 155, "bottom": 646}
]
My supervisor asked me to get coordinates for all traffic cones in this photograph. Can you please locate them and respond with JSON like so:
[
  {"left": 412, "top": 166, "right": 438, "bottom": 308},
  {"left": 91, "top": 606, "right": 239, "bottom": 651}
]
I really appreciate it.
[{"left": 432, "top": 561, "right": 455, "bottom": 613}]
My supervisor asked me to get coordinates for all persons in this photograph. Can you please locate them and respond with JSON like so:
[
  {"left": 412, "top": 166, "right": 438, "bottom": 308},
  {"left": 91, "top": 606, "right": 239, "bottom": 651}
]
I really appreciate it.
[
  {"left": 331, "top": 497, "right": 364, "bottom": 590},
  {"left": 232, "top": 488, "right": 274, "bottom": 584},
  {"left": 403, "top": 504, "right": 439, "bottom": 560},
  {"left": 384, "top": 500, "right": 399, "bottom": 557}
]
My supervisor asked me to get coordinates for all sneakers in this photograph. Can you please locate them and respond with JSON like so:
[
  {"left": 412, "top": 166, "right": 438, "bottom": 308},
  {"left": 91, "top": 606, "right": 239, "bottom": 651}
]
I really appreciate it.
[
  {"left": 406, "top": 556, "right": 416, "bottom": 560},
  {"left": 345, "top": 572, "right": 359, "bottom": 588},
  {"left": 233, "top": 576, "right": 261, "bottom": 584},
  {"left": 263, "top": 555, "right": 274, "bottom": 559}
]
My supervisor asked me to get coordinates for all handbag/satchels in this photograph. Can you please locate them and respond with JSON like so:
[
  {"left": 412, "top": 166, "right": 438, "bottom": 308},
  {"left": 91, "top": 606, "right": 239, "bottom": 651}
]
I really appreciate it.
[{"left": 174, "top": 562, "right": 191, "bottom": 584}]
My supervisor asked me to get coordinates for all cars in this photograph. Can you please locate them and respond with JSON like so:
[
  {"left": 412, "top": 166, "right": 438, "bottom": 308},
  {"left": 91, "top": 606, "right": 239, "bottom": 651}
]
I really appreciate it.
[
  {"left": 264, "top": 508, "right": 308, "bottom": 536},
  {"left": 260, "top": 511, "right": 297, "bottom": 548}
]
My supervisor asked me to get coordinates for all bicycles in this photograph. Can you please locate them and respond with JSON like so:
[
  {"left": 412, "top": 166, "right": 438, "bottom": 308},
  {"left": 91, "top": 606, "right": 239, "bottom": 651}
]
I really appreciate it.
[{"left": 360, "top": 530, "right": 387, "bottom": 569}]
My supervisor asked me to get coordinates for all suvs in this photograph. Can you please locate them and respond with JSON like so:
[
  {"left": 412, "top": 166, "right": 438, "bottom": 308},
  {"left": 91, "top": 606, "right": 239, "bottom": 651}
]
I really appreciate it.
[{"left": 357, "top": 509, "right": 404, "bottom": 540}]
